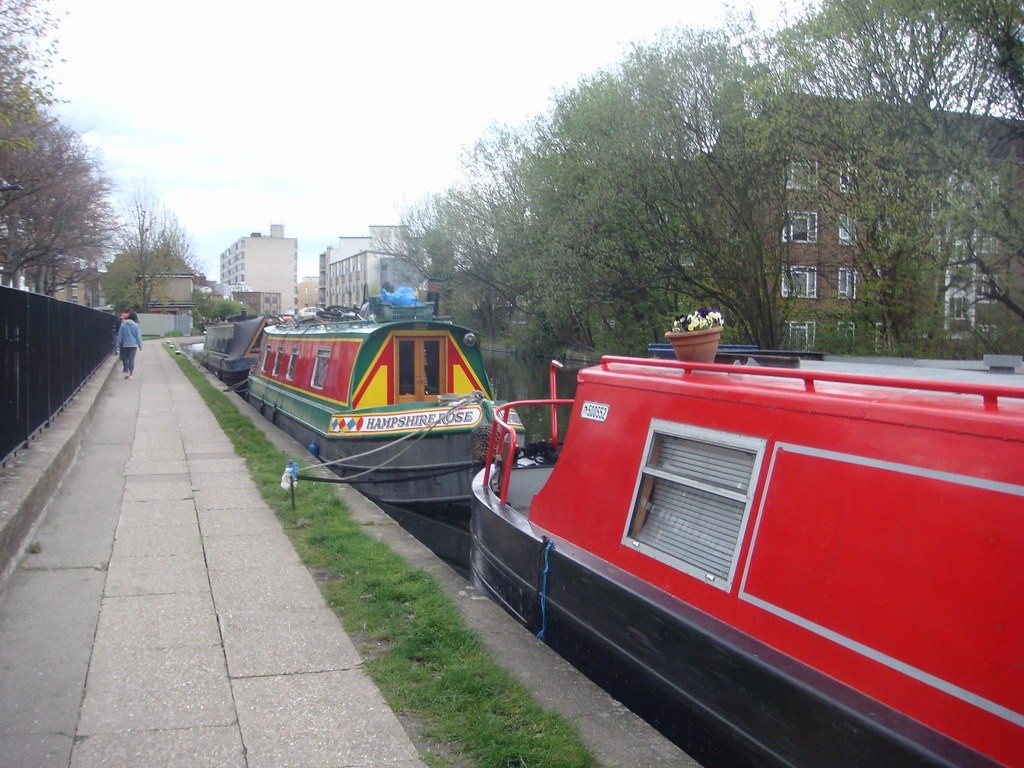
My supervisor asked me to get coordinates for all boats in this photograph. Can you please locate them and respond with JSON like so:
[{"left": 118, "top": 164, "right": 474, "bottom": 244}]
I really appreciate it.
[
  {"left": 192, "top": 308, "right": 296, "bottom": 392},
  {"left": 470, "top": 315, "right": 1024, "bottom": 768},
  {"left": 242, "top": 303, "right": 526, "bottom": 510}
]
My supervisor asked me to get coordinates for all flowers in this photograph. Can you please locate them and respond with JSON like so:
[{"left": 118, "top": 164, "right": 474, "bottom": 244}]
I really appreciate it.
[{"left": 670, "top": 305, "right": 725, "bottom": 331}]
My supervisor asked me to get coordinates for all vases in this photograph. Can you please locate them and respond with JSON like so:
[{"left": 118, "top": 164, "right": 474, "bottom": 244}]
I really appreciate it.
[{"left": 665, "top": 326, "right": 723, "bottom": 362}]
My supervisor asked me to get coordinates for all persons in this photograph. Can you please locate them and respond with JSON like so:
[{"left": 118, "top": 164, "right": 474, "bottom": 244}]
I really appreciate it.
[{"left": 114, "top": 308, "right": 143, "bottom": 380}]
